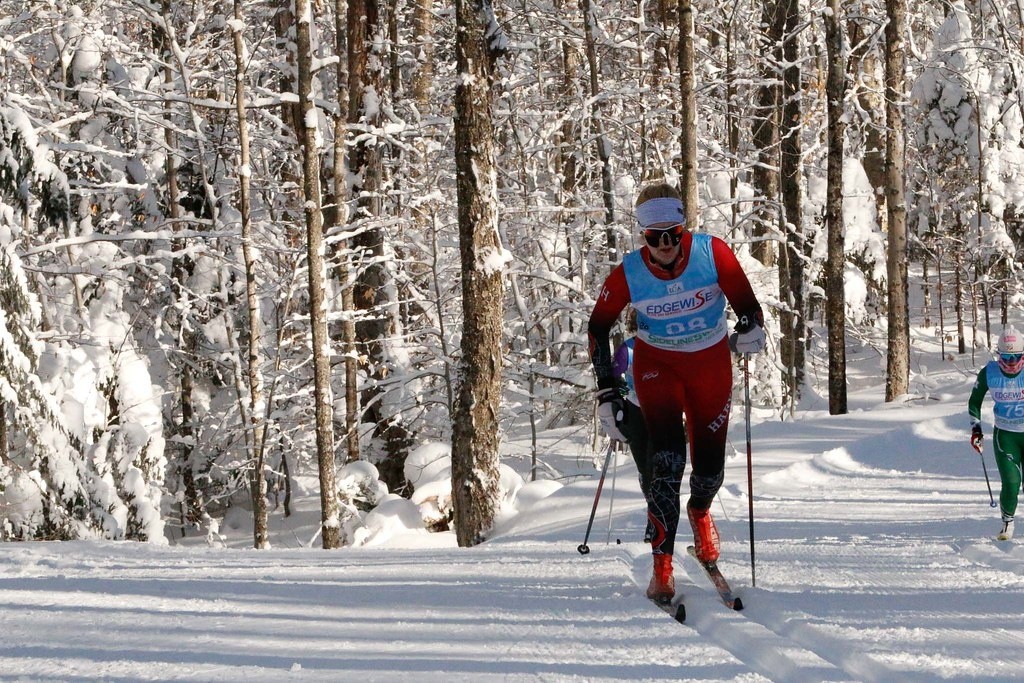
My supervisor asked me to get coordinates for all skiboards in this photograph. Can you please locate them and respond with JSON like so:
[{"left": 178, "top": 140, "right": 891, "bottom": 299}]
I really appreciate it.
[{"left": 643, "top": 544, "right": 744, "bottom": 622}]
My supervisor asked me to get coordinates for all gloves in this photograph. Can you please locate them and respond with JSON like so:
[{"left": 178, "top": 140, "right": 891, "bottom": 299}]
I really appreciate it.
[
  {"left": 971, "top": 432, "right": 983, "bottom": 454},
  {"left": 728, "top": 320, "right": 765, "bottom": 353},
  {"left": 596, "top": 386, "right": 639, "bottom": 443}
]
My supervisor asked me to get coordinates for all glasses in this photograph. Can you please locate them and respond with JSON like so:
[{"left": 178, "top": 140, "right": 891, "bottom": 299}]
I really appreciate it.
[
  {"left": 644, "top": 223, "right": 683, "bottom": 248},
  {"left": 1000, "top": 352, "right": 1024, "bottom": 362}
]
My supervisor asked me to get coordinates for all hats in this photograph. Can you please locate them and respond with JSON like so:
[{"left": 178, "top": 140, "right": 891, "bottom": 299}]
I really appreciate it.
[{"left": 998, "top": 325, "right": 1024, "bottom": 351}]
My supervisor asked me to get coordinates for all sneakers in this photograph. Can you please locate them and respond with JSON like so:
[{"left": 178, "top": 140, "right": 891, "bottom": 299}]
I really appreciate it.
[
  {"left": 647, "top": 553, "right": 675, "bottom": 602},
  {"left": 998, "top": 514, "right": 1014, "bottom": 540},
  {"left": 686, "top": 498, "right": 721, "bottom": 564}
]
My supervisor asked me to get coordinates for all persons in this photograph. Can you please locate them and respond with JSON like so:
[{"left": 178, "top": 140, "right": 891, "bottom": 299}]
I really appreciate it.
[
  {"left": 611, "top": 307, "right": 652, "bottom": 542},
  {"left": 587, "top": 184, "right": 767, "bottom": 605},
  {"left": 968, "top": 325, "right": 1024, "bottom": 539}
]
